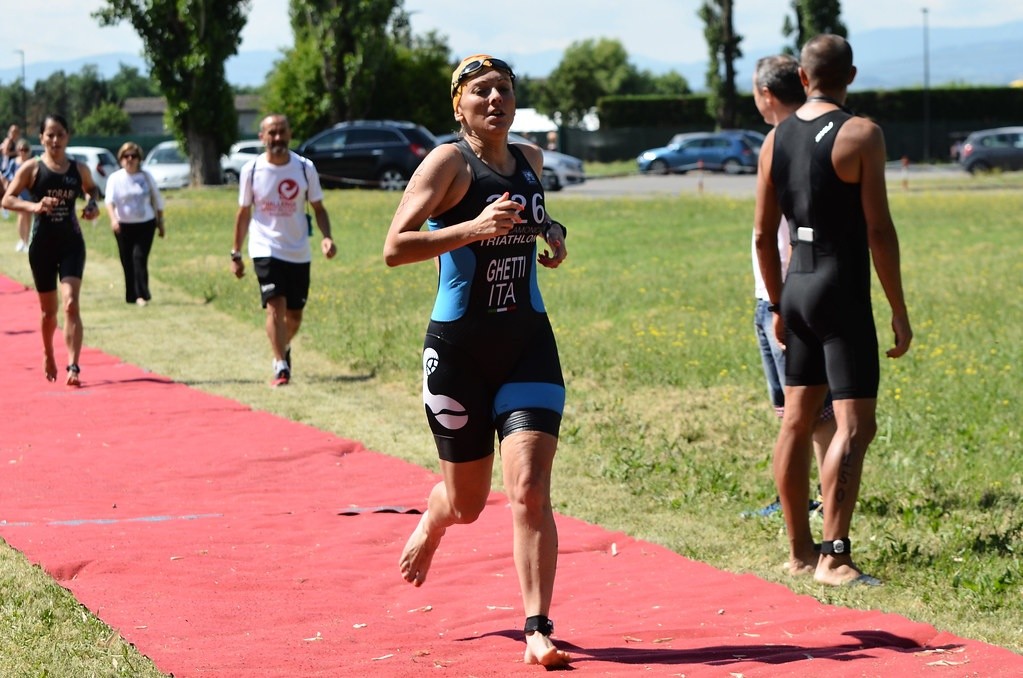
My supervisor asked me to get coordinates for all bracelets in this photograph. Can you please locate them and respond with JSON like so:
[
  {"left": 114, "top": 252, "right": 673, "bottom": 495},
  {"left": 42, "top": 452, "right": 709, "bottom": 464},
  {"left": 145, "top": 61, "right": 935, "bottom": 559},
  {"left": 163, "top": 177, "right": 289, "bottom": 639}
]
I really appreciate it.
[
  {"left": 768, "top": 303, "right": 780, "bottom": 312},
  {"left": 156, "top": 217, "right": 164, "bottom": 223}
]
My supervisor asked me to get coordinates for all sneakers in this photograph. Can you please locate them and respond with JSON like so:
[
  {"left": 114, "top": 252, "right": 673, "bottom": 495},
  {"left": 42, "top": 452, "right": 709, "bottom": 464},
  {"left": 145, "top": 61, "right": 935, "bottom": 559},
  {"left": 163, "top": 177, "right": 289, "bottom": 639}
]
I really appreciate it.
[
  {"left": 1, "top": 209, "right": 9, "bottom": 219},
  {"left": 272, "top": 369, "right": 291, "bottom": 386},
  {"left": 15, "top": 241, "right": 23, "bottom": 253},
  {"left": 285, "top": 348, "right": 292, "bottom": 368},
  {"left": 23, "top": 245, "right": 29, "bottom": 254}
]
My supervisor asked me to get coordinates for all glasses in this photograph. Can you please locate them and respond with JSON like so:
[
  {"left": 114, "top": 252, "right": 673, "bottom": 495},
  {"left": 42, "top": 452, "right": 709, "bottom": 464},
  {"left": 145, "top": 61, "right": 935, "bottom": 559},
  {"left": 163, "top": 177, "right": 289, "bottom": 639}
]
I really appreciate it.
[
  {"left": 121, "top": 153, "right": 141, "bottom": 159},
  {"left": 451, "top": 58, "right": 516, "bottom": 97},
  {"left": 16, "top": 147, "right": 30, "bottom": 153}
]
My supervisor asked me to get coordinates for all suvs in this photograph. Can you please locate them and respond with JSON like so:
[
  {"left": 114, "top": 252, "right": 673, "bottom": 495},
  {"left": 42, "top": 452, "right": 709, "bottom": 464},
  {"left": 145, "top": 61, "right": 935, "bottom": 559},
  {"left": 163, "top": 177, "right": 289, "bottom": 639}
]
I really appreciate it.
[{"left": 290, "top": 118, "right": 442, "bottom": 193}]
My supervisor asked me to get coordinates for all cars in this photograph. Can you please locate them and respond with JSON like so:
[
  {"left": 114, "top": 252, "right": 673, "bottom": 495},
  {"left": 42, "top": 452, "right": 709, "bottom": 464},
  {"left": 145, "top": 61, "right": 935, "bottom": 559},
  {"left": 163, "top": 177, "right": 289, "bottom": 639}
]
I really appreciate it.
[
  {"left": 438, "top": 132, "right": 588, "bottom": 192},
  {"left": 64, "top": 145, "right": 123, "bottom": 198},
  {"left": 959, "top": 126, "right": 1023, "bottom": 176},
  {"left": 139, "top": 140, "right": 191, "bottom": 191},
  {"left": 634, "top": 129, "right": 767, "bottom": 176},
  {"left": 218, "top": 139, "right": 267, "bottom": 186}
]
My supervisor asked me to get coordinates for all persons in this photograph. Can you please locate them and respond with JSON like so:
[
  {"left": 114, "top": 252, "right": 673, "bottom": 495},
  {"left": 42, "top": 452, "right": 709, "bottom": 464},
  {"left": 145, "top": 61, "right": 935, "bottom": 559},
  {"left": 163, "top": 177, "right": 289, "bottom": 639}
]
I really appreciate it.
[
  {"left": 740, "top": 52, "right": 825, "bottom": 525},
  {"left": 384, "top": 53, "right": 573, "bottom": 667},
  {"left": 0, "top": 125, "right": 34, "bottom": 255},
  {"left": 105, "top": 141, "right": 165, "bottom": 307},
  {"left": 231, "top": 114, "right": 336, "bottom": 385},
  {"left": 0, "top": 112, "right": 100, "bottom": 385},
  {"left": 753, "top": 35, "right": 914, "bottom": 589}
]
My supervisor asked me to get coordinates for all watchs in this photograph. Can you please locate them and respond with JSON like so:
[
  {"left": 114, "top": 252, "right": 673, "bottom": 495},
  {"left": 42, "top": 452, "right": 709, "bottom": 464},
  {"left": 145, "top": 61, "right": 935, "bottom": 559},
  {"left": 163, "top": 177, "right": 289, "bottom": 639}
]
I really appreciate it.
[
  {"left": 542, "top": 219, "right": 567, "bottom": 242},
  {"left": 231, "top": 251, "right": 242, "bottom": 261},
  {"left": 90, "top": 200, "right": 98, "bottom": 207}
]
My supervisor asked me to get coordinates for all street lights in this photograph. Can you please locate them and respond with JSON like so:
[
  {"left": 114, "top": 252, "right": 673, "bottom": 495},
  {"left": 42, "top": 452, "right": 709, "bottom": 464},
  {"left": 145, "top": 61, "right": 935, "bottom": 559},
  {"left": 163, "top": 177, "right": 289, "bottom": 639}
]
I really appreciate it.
[
  {"left": 10, "top": 47, "right": 27, "bottom": 139},
  {"left": 919, "top": 6, "right": 933, "bottom": 166}
]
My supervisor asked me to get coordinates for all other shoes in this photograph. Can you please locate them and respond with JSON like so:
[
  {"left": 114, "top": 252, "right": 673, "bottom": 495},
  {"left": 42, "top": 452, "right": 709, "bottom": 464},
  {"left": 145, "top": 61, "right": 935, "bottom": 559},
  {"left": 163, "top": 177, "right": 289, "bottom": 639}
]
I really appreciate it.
[
  {"left": 817, "top": 485, "right": 861, "bottom": 508},
  {"left": 136, "top": 298, "right": 147, "bottom": 308}
]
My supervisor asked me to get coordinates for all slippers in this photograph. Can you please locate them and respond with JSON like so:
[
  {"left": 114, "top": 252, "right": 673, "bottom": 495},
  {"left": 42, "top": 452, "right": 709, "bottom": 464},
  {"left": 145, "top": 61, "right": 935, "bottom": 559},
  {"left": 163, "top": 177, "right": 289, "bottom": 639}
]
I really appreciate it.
[{"left": 849, "top": 573, "right": 883, "bottom": 587}]
